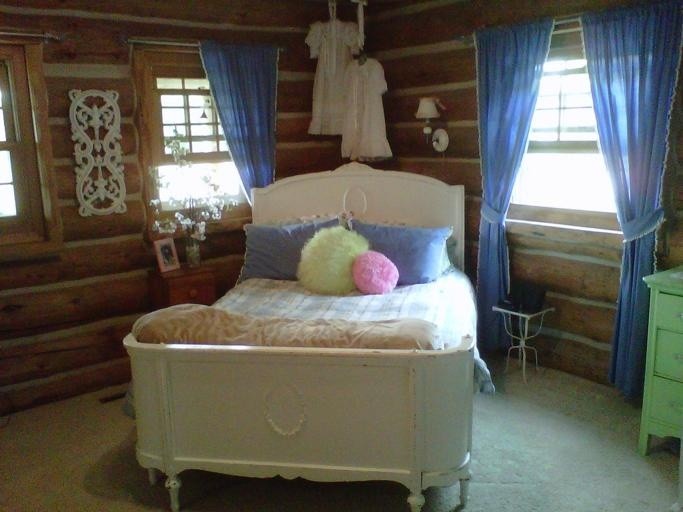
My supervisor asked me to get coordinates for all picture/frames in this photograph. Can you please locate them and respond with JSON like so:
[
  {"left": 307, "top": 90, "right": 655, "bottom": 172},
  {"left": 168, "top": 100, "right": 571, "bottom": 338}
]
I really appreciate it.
[{"left": 153, "top": 238, "right": 182, "bottom": 274}]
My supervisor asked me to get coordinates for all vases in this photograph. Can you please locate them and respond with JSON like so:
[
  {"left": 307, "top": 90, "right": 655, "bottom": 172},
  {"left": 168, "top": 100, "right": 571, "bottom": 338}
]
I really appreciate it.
[{"left": 185, "top": 237, "right": 203, "bottom": 271}]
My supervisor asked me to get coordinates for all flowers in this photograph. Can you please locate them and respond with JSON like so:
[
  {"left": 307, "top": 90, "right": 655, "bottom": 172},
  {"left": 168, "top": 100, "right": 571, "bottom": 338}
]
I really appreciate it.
[{"left": 148, "top": 126, "right": 238, "bottom": 242}]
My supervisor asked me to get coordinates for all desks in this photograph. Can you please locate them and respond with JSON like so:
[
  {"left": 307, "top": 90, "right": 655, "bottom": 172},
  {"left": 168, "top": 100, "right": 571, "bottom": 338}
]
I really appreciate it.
[{"left": 493, "top": 303, "right": 555, "bottom": 386}]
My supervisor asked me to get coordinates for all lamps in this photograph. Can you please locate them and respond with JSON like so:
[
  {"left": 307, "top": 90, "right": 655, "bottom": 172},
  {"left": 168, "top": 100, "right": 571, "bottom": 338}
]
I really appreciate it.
[{"left": 415, "top": 96, "right": 450, "bottom": 152}]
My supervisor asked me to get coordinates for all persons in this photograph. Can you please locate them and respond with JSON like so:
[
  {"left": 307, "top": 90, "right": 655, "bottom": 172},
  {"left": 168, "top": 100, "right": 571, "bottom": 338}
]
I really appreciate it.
[{"left": 163, "top": 245, "right": 173, "bottom": 265}]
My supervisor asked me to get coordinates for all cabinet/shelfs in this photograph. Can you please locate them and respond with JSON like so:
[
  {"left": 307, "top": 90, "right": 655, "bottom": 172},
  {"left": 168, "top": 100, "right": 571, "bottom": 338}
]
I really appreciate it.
[{"left": 637, "top": 265, "right": 683, "bottom": 478}]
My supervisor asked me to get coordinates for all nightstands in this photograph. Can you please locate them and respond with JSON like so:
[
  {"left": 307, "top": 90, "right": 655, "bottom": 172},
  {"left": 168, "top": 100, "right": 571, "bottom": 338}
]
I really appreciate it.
[
  {"left": 123, "top": 162, "right": 475, "bottom": 512},
  {"left": 148, "top": 270, "right": 216, "bottom": 309}
]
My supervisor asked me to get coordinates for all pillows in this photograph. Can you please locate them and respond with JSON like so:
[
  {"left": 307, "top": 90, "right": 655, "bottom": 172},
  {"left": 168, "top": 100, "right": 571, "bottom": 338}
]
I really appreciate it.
[
  {"left": 235, "top": 216, "right": 339, "bottom": 287},
  {"left": 346, "top": 218, "right": 454, "bottom": 285}
]
